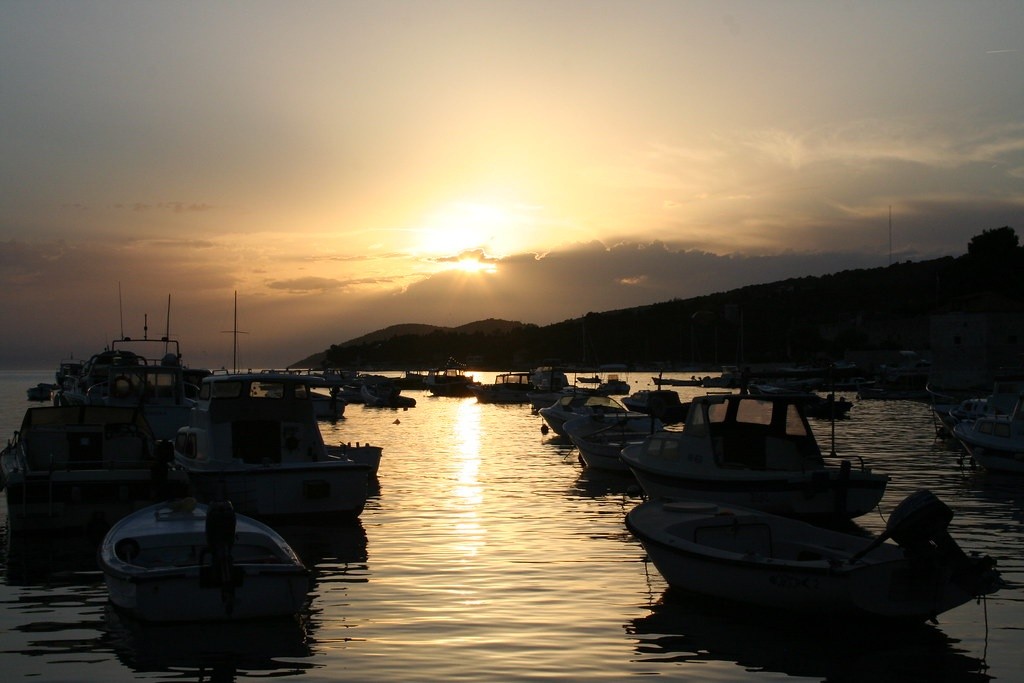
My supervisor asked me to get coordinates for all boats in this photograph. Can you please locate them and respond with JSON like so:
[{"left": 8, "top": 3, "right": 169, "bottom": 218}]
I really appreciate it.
[
  {"left": 615, "top": 389, "right": 894, "bottom": 527},
  {"left": 98, "top": 493, "right": 315, "bottom": 617},
  {"left": 618, "top": 491, "right": 1009, "bottom": 632},
  {"left": 0, "top": 279, "right": 1024, "bottom": 532}
]
